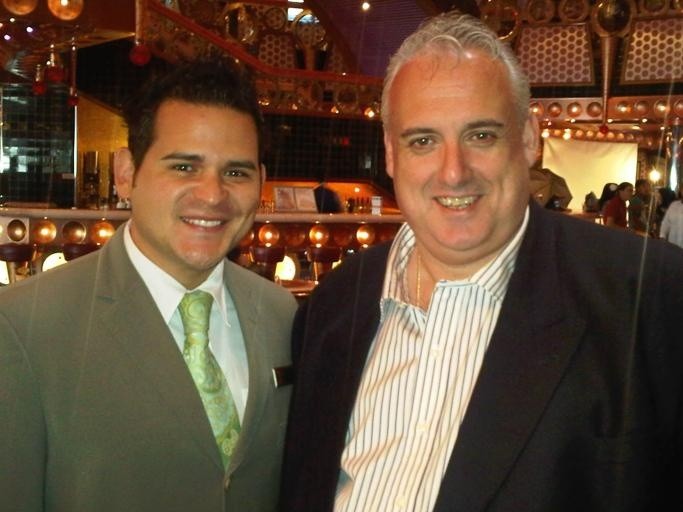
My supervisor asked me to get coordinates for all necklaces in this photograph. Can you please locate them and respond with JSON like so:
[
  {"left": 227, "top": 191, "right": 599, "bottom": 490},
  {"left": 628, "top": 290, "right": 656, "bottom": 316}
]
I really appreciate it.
[{"left": 415, "top": 247, "right": 424, "bottom": 309}]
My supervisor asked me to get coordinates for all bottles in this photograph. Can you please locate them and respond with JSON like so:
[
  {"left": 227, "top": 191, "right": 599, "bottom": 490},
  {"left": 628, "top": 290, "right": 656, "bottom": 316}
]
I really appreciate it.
[{"left": 83, "top": 151, "right": 118, "bottom": 204}]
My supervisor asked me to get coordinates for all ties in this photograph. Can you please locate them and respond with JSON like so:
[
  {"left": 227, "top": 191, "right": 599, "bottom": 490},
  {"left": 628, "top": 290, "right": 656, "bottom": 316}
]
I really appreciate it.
[{"left": 177, "top": 291, "right": 241, "bottom": 474}]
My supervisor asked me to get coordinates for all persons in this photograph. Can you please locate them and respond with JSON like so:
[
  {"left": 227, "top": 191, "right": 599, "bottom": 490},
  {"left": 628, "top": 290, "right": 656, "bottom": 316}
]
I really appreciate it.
[
  {"left": 629, "top": 180, "right": 654, "bottom": 238},
  {"left": 659, "top": 192, "right": 683, "bottom": 249},
  {"left": 285, "top": 7, "right": 683, "bottom": 512},
  {"left": 651, "top": 189, "right": 675, "bottom": 240},
  {"left": 1, "top": 52, "right": 299, "bottom": 512},
  {"left": 603, "top": 181, "right": 633, "bottom": 232}
]
